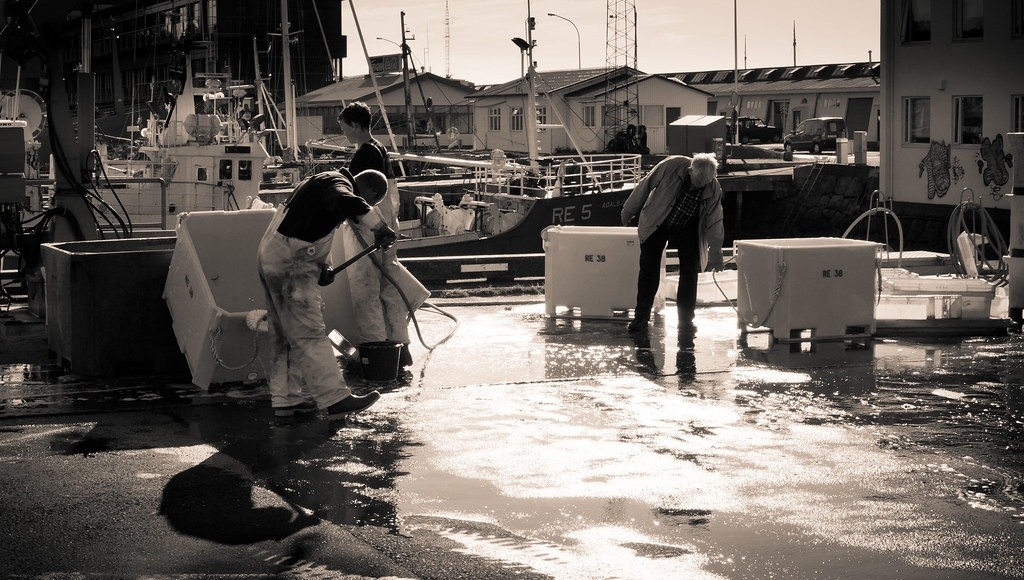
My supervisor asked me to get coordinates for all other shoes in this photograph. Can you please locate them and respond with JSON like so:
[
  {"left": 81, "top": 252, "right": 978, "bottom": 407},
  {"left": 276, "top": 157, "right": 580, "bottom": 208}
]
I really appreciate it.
[
  {"left": 629, "top": 317, "right": 648, "bottom": 330},
  {"left": 678, "top": 307, "right": 695, "bottom": 320}
]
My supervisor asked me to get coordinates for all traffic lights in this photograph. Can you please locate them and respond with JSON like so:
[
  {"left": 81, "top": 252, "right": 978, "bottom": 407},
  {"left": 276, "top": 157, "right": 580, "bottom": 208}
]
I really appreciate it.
[{"left": 530, "top": 16, "right": 535, "bottom": 31}]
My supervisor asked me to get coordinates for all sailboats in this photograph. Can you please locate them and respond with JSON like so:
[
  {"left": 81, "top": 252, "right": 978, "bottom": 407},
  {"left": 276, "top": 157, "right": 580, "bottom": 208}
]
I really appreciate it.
[{"left": 0, "top": 0, "right": 882, "bottom": 251}]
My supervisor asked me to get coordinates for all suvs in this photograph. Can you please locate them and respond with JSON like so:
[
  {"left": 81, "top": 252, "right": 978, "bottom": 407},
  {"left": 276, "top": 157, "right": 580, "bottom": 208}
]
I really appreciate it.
[
  {"left": 725, "top": 116, "right": 784, "bottom": 144},
  {"left": 783, "top": 116, "right": 850, "bottom": 155}
]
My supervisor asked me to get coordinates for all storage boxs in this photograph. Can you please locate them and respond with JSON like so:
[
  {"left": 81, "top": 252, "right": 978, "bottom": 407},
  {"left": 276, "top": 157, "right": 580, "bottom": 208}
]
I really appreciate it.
[
  {"left": 162, "top": 207, "right": 273, "bottom": 390},
  {"left": 1002, "top": 132, "right": 1024, "bottom": 319},
  {"left": 735, "top": 237, "right": 882, "bottom": 340},
  {"left": 541, "top": 223, "right": 668, "bottom": 317},
  {"left": 876, "top": 293, "right": 995, "bottom": 321}
]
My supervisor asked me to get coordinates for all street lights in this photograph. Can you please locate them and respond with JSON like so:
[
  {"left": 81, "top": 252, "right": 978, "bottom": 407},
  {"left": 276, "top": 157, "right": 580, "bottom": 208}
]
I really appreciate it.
[
  {"left": 547, "top": 13, "right": 581, "bottom": 70},
  {"left": 375, "top": 37, "right": 405, "bottom": 71},
  {"left": 510, "top": 36, "right": 538, "bottom": 169}
]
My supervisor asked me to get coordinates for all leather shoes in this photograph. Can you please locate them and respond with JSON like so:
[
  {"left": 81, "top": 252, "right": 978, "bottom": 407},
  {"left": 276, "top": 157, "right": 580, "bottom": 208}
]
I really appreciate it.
[
  {"left": 275, "top": 401, "right": 316, "bottom": 416},
  {"left": 327, "top": 391, "right": 380, "bottom": 420}
]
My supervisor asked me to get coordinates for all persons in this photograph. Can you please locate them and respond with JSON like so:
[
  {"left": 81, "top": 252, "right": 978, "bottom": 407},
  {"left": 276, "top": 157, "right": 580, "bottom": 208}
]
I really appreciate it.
[
  {"left": 338, "top": 102, "right": 409, "bottom": 346},
  {"left": 256, "top": 168, "right": 398, "bottom": 420},
  {"left": 621, "top": 153, "right": 724, "bottom": 331}
]
